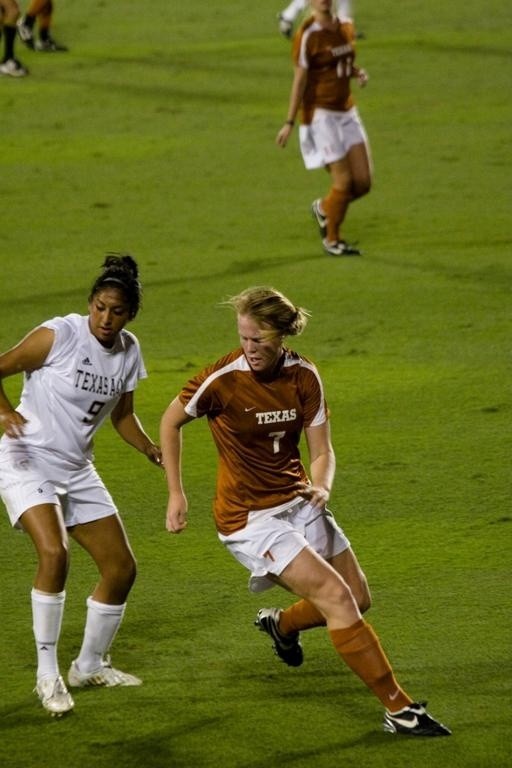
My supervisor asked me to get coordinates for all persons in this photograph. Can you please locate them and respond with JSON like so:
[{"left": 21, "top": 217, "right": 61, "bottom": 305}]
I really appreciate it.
[
  {"left": 16, "top": 1, "right": 68, "bottom": 52},
  {"left": 0, "top": 0, "right": 29, "bottom": 77},
  {"left": 165, "top": 288, "right": 452, "bottom": 738},
  {"left": 276, "top": 3, "right": 365, "bottom": 41},
  {"left": 277, "top": 1, "right": 376, "bottom": 254},
  {"left": 2, "top": 252, "right": 162, "bottom": 717}
]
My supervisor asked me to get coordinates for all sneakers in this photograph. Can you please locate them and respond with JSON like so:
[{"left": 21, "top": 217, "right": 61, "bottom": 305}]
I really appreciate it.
[
  {"left": 323, "top": 239, "right": 360, "bottom": 256},
  {"left": 254, "top": 607, "right": 303, "bottom": 667},
  {"left": 36, "top": 675, "right": 75, "bottom": 714},
  {"left": 382, "top": 700, "right": 452, "bottom": 736},
  {"left": 0, "top": 17, "right": 68, "bottom": 77},
  {"left": 68, "top": 660, "right": 143, "bottom": 688},
  {"left": 312, "top": 199, "right": 327, "bottom": 239}
]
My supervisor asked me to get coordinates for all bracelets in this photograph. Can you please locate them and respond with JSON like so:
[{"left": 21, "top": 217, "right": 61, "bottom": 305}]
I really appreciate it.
[{"left": 286, "top": 119, "right": 294, "bottom": 127}]
